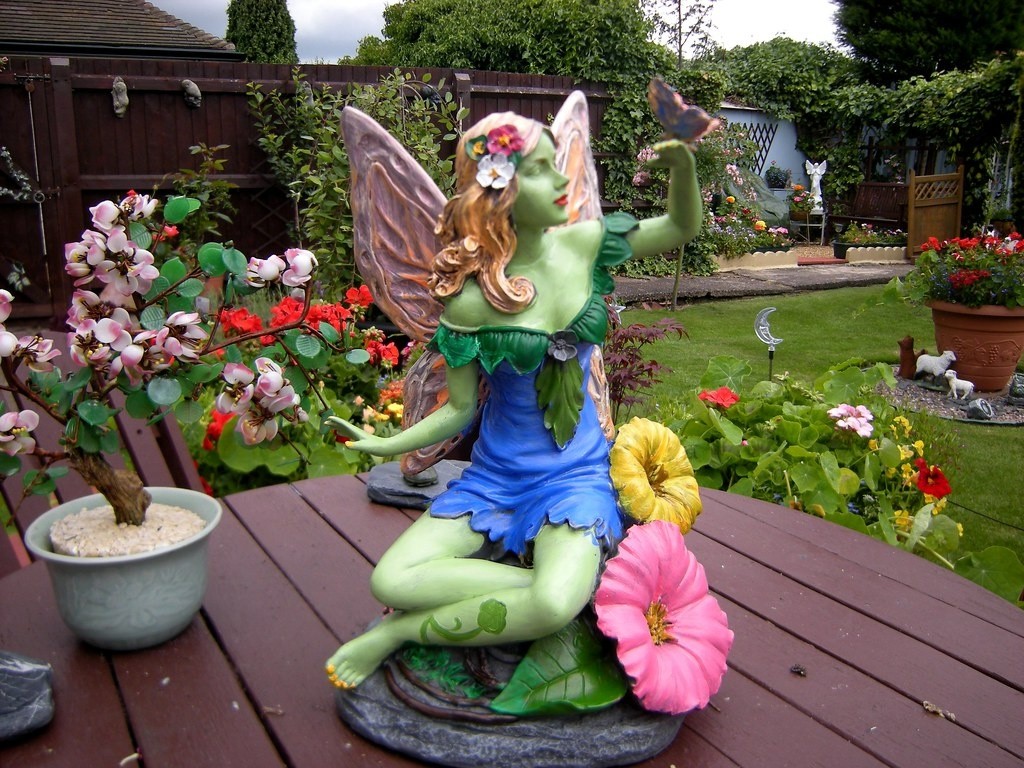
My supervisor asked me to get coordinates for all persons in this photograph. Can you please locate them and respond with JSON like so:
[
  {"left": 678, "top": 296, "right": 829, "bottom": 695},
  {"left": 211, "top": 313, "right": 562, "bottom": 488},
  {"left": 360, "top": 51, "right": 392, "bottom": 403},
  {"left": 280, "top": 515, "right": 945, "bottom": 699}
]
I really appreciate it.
[
  {"left": 811, "top": 163, "right": 822, "bottom": 206},
  {"left": 325, "top": 111, "right": 703, "bottom": 689}
]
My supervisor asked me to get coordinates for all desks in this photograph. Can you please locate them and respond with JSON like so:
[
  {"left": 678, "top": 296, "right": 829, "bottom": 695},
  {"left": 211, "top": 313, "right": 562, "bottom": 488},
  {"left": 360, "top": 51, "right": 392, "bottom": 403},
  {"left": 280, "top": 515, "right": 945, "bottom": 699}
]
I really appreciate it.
[{"left": 0, "top": 472, "right": 1024, "bottom": 768}]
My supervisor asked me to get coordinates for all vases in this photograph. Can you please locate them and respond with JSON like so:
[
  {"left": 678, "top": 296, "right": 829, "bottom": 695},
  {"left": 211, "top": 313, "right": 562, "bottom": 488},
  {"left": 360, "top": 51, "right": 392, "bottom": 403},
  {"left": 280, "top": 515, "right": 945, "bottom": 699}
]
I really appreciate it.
[
  {"left": 924, "top": 299, "right": 1024, "bottom": 393},
  {"left": 24, "top": 488, "right": 223, "bottom": 653},
  {"left": 832, "top": 242, "right": 906, "bottom": 259},
  {"left": 767, "top": 181, "right": 785, "bottom": 189},
  {"left": 789, "top": 210, "right": 808, "bottom": 222}
]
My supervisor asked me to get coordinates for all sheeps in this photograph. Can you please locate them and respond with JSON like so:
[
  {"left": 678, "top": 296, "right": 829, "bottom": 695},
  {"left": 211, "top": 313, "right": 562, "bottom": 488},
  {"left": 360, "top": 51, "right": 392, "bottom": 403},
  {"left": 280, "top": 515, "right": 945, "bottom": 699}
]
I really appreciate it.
[{"left": 913, "top": 350, "right": 974, "bottom": 400}]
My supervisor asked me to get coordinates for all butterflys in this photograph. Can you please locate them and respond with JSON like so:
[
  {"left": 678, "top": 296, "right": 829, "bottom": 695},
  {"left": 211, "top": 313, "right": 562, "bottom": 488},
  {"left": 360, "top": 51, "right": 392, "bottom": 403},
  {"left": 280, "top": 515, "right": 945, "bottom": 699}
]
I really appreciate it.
[{"left": 647, "top": 76, "right": 720, "bottom": 152}]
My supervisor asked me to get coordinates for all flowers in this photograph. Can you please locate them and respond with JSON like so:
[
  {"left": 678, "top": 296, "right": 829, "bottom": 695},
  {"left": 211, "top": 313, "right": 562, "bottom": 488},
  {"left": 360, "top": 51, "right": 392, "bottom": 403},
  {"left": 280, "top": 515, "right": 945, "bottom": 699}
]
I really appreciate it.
[
  {"left": 0, "top": 188, "right": 375, "bottom": 535},
  {"left": 830, "top": 220, "right": 908, "bottom": 245},
  {"left": 764, "top": 160, "right": 793, "bottom": 186},
  {"left": 487, "top": 519, "right": 736, "bottom": 716},
  {"left": 464, "top": 124, "right": 527, "bottom": 191},
  {"left": 875, "top": 223, "right": 1024, "bottom": 310},
  {"left": 783, "top": 176, "right": 822, "bottom": 214},
  {"left": 609, "top": 416, "right": 703, "bottom": 535},
  {"left": 675, "top": 355, "right": 1024, "bottom": 606}
]
